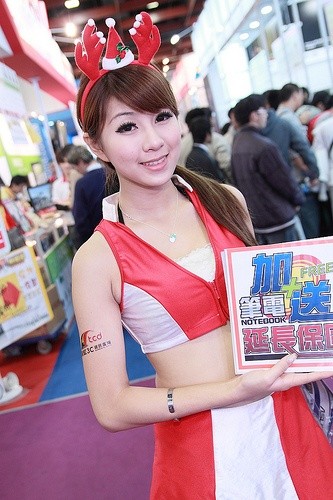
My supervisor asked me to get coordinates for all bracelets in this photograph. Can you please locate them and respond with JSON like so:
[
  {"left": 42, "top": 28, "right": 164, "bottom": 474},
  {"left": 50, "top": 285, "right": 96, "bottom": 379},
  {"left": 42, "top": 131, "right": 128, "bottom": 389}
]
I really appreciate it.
[{"left": 167, "top": 387, "right": 179, "bottom": 422}]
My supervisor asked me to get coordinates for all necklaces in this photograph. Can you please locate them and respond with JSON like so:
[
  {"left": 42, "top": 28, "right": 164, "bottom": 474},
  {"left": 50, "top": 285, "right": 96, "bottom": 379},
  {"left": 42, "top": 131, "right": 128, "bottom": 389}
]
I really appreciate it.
[{"left": 117, "top": 186, "right": 178, "bottom": 242}]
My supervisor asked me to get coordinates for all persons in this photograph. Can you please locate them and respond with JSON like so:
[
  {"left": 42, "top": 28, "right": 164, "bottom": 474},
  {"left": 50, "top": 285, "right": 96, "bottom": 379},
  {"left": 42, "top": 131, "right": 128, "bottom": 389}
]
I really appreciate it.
[
  {"left": 4, "top": 83, "right": 333, "bottom": 251},
  {"left": 68, "top": 54, "right": 333, "bottom": 500}
]
